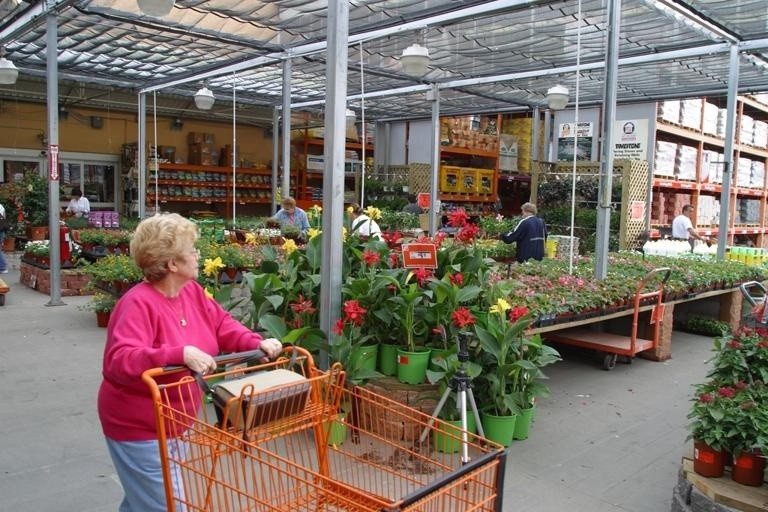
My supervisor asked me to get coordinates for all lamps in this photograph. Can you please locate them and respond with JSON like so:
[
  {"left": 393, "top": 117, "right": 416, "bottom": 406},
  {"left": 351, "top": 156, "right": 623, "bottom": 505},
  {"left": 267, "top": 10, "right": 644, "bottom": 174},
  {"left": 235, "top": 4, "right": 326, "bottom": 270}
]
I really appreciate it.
[
  {"left": 401, "top": 43, "right": 425, "bottom": 73},
  {"left": 192, "top": 88, "right": 215, "bottom": 112},
  {"left": 1, "top": 59, "right": 19, "bottom": 86},
  {"left": 546, "top": 84, "right": 568, "bottom": 107},
  {"left": 137, "top": 1, "right": 174, "bottom": 18}
]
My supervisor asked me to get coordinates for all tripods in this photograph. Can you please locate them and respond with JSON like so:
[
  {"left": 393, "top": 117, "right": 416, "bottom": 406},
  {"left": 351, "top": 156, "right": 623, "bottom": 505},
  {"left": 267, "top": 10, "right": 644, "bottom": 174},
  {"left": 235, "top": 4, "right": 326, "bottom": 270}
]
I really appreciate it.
[{"left": 418, "top": 331, "right": 488, "bottom": 466}]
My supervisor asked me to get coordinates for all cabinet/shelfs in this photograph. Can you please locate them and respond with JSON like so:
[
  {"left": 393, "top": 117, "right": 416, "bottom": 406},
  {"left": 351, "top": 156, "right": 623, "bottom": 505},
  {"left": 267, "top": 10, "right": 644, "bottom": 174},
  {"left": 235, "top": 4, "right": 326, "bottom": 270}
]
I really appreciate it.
[
  {"left": 146, "top": 144, "right": 298, "bottom": 218},
  {"left": 649, "top": 89, "right": 768, "bottom": 251},
  {"left": 440, "top": 113, "right": 504, "bottom": 219},
  {"left": 290, "top": 121, "right": 373, "bottom": 211}
]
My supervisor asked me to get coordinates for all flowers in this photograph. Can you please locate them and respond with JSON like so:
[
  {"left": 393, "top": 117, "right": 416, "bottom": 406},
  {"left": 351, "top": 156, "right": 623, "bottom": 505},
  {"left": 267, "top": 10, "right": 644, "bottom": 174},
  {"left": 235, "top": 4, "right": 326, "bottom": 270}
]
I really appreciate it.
[
  {"left": 534, "top": 173, "right": 622, "bottom": 254},
  {"left": 480, "top": 248, "right": 768, "bottom": 317},
  {"left": 67, "top": 200, "right": 383, "bottom": 312},
  {"left": 683, "top": 310, "right": 767, "bottom": 450},
  {"left": 230, "top": 200, "right": 563, "bottom": 354}
]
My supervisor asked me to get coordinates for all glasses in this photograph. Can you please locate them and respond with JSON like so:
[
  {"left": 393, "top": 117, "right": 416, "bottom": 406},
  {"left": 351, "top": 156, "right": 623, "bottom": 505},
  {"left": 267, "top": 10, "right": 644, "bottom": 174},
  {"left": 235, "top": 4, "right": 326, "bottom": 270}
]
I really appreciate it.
[{"left": 178, "top": 248, "right": 203, "bottom": 258}]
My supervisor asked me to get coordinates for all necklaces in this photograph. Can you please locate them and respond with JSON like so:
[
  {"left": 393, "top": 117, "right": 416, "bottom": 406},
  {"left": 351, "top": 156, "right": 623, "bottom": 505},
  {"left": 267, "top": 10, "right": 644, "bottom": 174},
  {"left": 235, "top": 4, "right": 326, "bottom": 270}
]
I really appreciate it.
[{"left": 156, "top": 283, "right": 189, "bottom": 328}]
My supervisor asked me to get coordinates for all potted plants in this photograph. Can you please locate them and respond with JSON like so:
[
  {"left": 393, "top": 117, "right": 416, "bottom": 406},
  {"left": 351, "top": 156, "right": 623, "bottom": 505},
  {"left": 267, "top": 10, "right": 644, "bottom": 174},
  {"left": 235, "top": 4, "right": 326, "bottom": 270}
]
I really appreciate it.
[
  {"left": 0, "top": 174, "right": 48, "bottom": 252},
  {"left": 357, "top": 174, "right": 411, "bottom": 211}
]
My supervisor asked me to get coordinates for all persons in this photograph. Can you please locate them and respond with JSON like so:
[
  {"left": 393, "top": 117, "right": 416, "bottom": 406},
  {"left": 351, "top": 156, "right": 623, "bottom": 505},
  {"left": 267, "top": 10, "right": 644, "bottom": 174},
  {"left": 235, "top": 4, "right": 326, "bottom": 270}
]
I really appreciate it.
[
  {"left": 272, "top": 197, "right": 311, "bottom": 239},
  {"left": 97, "top": 212, "right": 282, "bottom": 512},
  {"left": 672, "top": 205, "right": 709, "bottom": 242},
  {"left": 0, "top": 204, "right": 8, "bottom": 274},
  {"left": 500, "top": 203, "right": 548, "bottom": 263},
  {"left": 402, "top": 194, "right": 423, "bottom": 214},
  {"left": 66, "top": 188, "right": 90, "bottom": 219},
  {"left": 349, "top": 202, "right": 384, "bottom": 241}
]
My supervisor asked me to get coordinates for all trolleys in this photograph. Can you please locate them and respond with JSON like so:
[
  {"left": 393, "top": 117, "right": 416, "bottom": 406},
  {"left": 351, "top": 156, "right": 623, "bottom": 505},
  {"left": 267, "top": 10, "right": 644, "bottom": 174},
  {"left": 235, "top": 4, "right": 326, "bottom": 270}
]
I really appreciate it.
[
  {"left": 543, "top": 266, "right": 670, "bottom": 372},
  {"left": 142, "top": 345, "right": 507, "bottom": 512}
]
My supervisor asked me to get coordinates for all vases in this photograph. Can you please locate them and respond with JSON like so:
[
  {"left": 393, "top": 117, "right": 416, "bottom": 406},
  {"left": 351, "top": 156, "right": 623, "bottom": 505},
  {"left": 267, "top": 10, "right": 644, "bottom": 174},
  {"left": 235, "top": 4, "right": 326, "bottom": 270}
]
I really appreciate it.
[
  {"left": 481, "top": 406, "right": 515, "bottom": 449},
  {"left": 376, "top": 344, "right": 398, "bottom": 376},
  {"left": 120, "top": 282, "right": 135, "bottom": 293},
  {"left": 694, "top": 440, "right": 726, "bottom": 476},
  {"left": 450, "top": 412, "right": 476, "bottom": 443},
  {"left": 396, "top": 349, "right": 430, "bottom": 384},
  {"left": 347, "top": 344, "right": 377, "bottom": 376},
  {"left": 82, "top": 243, "right": 94, "bottom": 251},
  {"left": 119, "top": 244, "right": 128, "bottom": 251},
  {"left": 25, "top": 254, "right": 48, "bottom": 269},
  {"left": 432, "top": 418, "right": 468, "bottom": 452},
  {"left": 239, "top": 266, "right": 247, "bottom": 273},
  {"left": 515, "top": 404, "right": 534, "bottom": 440},
  {"left": 318, "top": 399, "right": 348, "bottom": 446},
  {"left": 248, "top": 354, "right": 267, "bottom": 370},
  {"left": 269, "top": 222, "right": 279, "bottom": 228},
  {"left": 503, "top": 257, "right": 512, "bottom": 264},
  {"left": 112, "top": 280, "right": 118, "bottom": 291},
  {"left": 101, "top": 280, "right": 108, "bottom": 289},
  {"left": 226, "top": 269, "right": 235, "bottom": 277},
  {"left": 429, "top": 348, "right": 453, "bottom": 370},
  {"left": 97, "top": 311, "right": 111, "bottom": 326},
  {"left": 202, "top": 371, "right": 224, "bottom": 403},
  {"left": 731, "top": 450, "right": 765, "bottom": 487}
]
